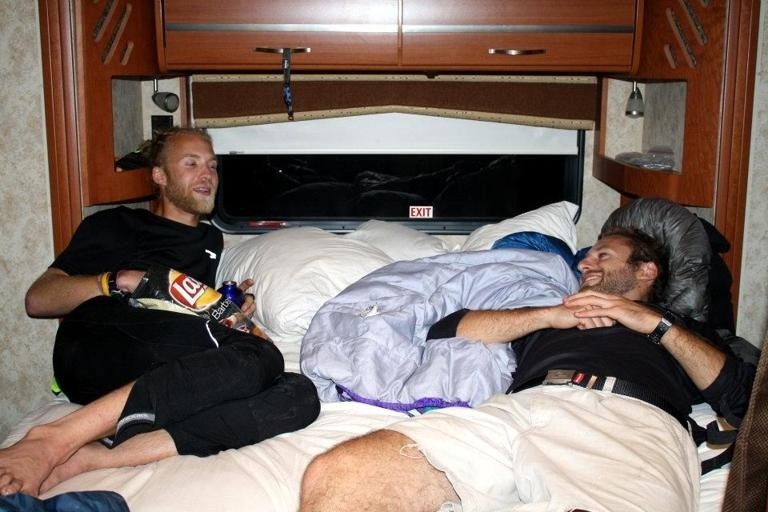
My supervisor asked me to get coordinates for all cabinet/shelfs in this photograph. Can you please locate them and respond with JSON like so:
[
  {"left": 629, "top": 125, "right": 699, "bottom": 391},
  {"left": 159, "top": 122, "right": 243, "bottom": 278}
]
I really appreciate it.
[{"left": 154, "top": 0, "right": 653, "bottom": 77}]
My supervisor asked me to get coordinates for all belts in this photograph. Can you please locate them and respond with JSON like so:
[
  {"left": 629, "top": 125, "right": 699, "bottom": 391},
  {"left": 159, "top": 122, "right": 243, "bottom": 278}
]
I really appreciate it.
[{"left": 514, "top": 367, "right": 689, "bottom": 432}]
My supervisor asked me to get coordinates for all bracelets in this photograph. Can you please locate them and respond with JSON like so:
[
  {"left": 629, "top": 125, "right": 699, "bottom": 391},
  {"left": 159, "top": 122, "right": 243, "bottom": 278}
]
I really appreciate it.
[
  {"left": 101, "top": 271, "right": 111, "bottom": 297},
  {"left": 648, "top": 311, "right": 676, "bottom": 344},
  {"left": 109, "top": 268, "right": 125, "bottom": 299}
]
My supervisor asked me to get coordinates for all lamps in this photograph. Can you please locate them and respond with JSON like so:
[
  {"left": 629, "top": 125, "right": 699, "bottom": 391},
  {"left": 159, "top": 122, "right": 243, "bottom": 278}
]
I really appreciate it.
[
  {"left": 625, "top": 79, "right": 646, "bottom": 117},
  {"left": 150, "top": 76, "right": 182, "bottom": 114}
]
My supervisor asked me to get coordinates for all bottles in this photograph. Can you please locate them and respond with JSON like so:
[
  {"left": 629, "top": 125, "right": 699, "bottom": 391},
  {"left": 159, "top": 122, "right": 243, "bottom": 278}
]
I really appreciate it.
[{"left": 217, "top": 281, "right": 245, "bottom": 310}]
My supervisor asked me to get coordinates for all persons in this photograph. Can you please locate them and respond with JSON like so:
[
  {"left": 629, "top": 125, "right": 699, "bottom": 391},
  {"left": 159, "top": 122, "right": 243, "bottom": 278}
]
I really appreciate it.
[
  {"left": 297, "top": 225, "right": 759, "bottom": 512},
  {"left": 1, "top": 124, "right": 321, "bottom": 498}
]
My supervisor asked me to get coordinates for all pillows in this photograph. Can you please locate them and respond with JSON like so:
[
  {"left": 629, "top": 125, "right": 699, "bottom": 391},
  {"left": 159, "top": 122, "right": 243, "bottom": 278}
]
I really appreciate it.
[{"left": 216, "top": 198, "right": 623, "bottom": 351}]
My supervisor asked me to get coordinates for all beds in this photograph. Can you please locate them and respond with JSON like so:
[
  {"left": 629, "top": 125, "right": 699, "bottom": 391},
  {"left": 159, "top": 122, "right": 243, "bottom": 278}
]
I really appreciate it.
[{"left": 0, "top": 103, "right": 738, "bottom": 512}]
